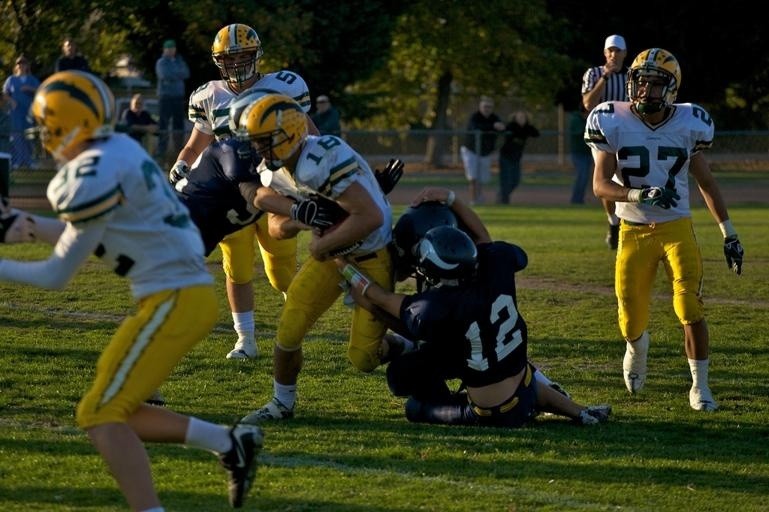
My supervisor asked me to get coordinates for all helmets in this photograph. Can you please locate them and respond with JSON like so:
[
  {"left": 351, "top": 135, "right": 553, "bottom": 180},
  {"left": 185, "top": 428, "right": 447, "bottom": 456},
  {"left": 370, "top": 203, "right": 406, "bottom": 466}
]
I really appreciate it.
[
  {"left": 402, "top": 225, "right": 480, "bottom": 290},
  {"left": 394, "top": 200, "right": 458, "bottom": 282},
  {"left": 23, "top": 69, "right": 118, "bottom": 169},
  {"left": 624, "top": 49, "right": 682, "bottom": 120},
  {"left": 211, "top": 23, "right": 308, "bottom": 173}
]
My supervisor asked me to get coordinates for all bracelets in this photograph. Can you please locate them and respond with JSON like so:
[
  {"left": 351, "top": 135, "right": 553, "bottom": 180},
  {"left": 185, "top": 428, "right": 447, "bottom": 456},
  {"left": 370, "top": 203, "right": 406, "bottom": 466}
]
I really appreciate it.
[
  {"left": 627, "top": 188, "right": 642, "bottom": 203},
  {"left": 719, "top": 218, "right": 737, "bottom": 240}
]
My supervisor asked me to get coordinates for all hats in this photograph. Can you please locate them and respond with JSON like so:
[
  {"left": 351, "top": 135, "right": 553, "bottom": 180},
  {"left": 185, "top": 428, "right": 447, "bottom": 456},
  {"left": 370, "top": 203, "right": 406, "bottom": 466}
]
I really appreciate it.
[{"left": 604, "top": 34, "right": 626, "bottom": 50}]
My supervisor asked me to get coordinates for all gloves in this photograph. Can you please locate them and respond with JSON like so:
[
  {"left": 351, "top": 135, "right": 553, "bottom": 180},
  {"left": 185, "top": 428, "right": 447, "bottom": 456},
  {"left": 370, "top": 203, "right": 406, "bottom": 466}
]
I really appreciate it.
[
  {"left": 169, "top": 160, "right": 189, "bottom": 185},
  {"left": 723, "top": 234, "right": 743, "bottom": 275},
  {"left": 374, "top": 158, "right": 405, "bottom": 194},
  {"left": 293, "top": 193, "right": 334, "bottom": 230},
  {"left": 637, "top": 186, "right": 681, "bottom": 210}
]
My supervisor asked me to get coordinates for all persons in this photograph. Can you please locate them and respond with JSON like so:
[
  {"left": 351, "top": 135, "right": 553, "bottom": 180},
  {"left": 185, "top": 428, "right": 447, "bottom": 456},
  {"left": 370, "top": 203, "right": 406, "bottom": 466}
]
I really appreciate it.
[
  {"left": 53, "top": 37, "right": 92, "bottom": 73},
  {"left": 570, "top": 108, "right": 595, "bottom": 205},
  {"left": 120, "top": 93, "right": 153, "bottom": 141},
  {"left": 584, "top": 48, "right": 744, "bottom": 411},
  {"left": 2, "top": 56, "right": 42, "bottom": 170},
  {"left": 579, "top": 34, "right": 630, "bottom": 251},
  {"left": 0, "top": 69, "right": 265, "bottom": 512},
  {"left": 331, "top": 187, "right": 610, "bottom": 427},
  {"left": 155, "top": 24, "right": 412, "bottom": 430},
  {"left": 461, "top": 97, "right": 540, "bottom": 204}
]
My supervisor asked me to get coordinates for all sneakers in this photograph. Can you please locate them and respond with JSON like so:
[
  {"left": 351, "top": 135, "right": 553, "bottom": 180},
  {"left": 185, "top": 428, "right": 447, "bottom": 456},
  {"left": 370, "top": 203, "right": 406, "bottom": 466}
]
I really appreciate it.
[
  {"left": 226, "top": 336, "right": 258, "bottom": 360},
  {"left": 622, "top": 331, "right": 649, "bottom": 393},
  {"left": 574, "top": 404, "right": 612, "bottom": 428},
  {"left": 605, "top": 223, "right": 619, "bottom": 250},
  {"left": 218, "top": 423, "right": 265, "bottom": 508},
  {"left": 241, "top": 397, "right": 296, "bottom": 424},
  {"left": 688, "top": 385, "right": 717, "bottom": 411}
]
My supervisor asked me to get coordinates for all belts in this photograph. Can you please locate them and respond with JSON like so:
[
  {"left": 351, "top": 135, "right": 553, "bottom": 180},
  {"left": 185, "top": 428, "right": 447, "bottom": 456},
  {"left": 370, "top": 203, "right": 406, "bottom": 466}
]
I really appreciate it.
[{"left": 466, "top": 364, "right": 531, "bottom": 417}]
